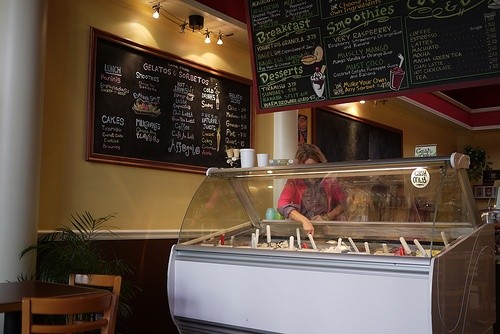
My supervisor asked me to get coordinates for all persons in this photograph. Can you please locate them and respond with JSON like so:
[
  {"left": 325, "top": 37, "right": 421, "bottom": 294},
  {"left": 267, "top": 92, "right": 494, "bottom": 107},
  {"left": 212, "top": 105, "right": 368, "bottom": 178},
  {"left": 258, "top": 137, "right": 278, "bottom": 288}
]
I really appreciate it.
[{"left": 277, "top": 142, "right": 348, "bottom": 236}]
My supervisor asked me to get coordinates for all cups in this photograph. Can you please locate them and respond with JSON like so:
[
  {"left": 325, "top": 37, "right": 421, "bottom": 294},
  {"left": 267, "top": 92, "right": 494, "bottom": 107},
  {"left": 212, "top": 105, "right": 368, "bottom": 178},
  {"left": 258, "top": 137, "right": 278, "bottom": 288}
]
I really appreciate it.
[
  {"left": 240, "top": 149, "right": 255, "bottom": 168},
  {"left": 265, "top": 208, "right": 275, "bottom": 220},
  {"left": 257, "top": 154, "right": 268, "bottom": 167},
  {"left": 275, "top": 211, "right": 285, "bottom": 220}
]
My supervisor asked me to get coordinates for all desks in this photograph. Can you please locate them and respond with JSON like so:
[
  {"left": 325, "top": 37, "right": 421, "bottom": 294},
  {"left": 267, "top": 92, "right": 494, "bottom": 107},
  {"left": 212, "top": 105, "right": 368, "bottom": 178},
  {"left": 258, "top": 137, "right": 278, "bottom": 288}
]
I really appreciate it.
[{"left": 0, "top": 280, "right": 110, "bottom": 334}]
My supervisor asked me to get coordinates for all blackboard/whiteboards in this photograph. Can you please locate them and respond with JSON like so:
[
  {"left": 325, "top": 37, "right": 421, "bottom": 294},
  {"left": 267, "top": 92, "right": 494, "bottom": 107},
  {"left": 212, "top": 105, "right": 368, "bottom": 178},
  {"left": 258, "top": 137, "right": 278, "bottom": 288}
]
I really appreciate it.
[
  {"left": 245, "top": 1, "right": 500, "bottom": 114},
  {"left": 85, "top": 25, "right": 255, "bottom": 179}
]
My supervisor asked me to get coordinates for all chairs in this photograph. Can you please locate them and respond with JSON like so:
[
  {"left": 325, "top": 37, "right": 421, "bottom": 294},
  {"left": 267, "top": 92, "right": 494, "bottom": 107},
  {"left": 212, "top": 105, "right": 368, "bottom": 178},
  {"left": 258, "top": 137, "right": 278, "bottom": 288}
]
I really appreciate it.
[
  {"left": 65, "top": 273, "right": 122, "bottom": 334},
  {"left": 21, "top": 293, "right": 117, "bottom": 334}
]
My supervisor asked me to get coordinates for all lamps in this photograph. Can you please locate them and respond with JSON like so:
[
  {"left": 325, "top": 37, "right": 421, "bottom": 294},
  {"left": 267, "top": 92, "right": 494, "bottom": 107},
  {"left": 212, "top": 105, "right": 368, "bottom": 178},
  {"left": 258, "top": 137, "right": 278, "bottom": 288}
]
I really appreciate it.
[
  {"left": 204, "top": 28, "right": 212, "bottom": 43},
  {"left": 217, "top": 32, "right": 223, "bottom": 45},
  {"left": 179, "top": 20, "right": 188, "bottom": 33},
  {"left": 152, "top": 2, "right": 161, "bottom": 18}
]
